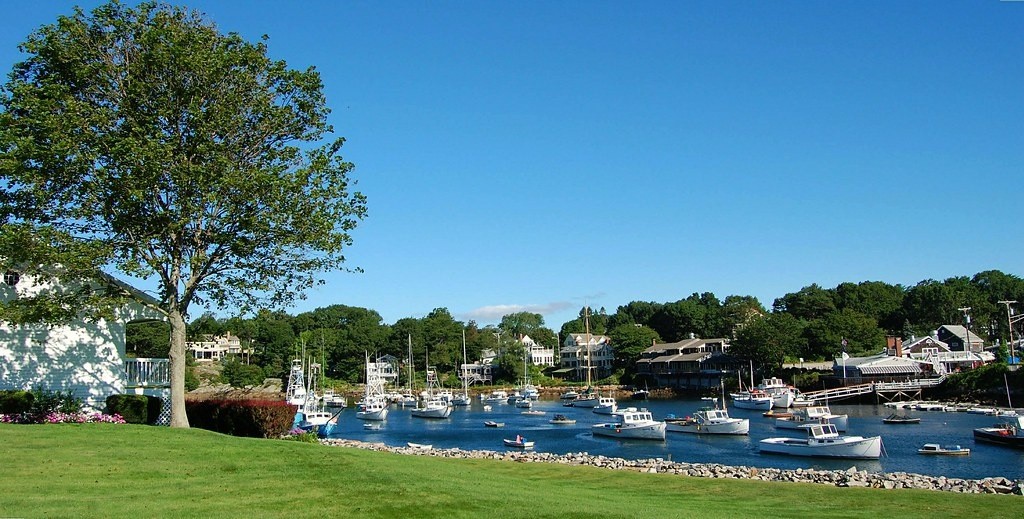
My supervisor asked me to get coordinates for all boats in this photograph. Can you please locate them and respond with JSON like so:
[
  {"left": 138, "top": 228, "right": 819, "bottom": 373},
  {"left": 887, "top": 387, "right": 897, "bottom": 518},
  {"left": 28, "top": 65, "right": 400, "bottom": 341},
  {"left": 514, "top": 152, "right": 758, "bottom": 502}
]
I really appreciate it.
[
  {"left": 729, "top": 369, "right": 774, "bottom": 411},
  {"left": 995, "top": 373, "right": 1019, "bottom": 423},
  {"left": 287, "top": 301, "right": 599, "bottom": 452},
  {"left": 762, "top": 410, "right": 794, "bottom": 417},
  {"left": 503, "top": 434, "right": 535, "bottom": 447},
  {"left": 520, "top": 410, "right": 546, "bottom": 415},
  {"left": 592, "top": 397, "right": 618, "bottom": 415},
  {"left": 754, "top": 376, "right": 796, "bottom": 408},
  {"left": 916, "top": 443, "right": 970, "bottom": 457},
  {"left": 758, "top": 423, "right": 882, "bottom": 460},
  {"left": 663, "top": 378, "right": 750, "bottom": 437},
  {"left": 973, "top": 421, "right": 1024, "bottom": 448},
  {"left": 883, "top": 415, "right": 921, "bottom": 424},
  {"left": 548, "top": 413, "right": 577, "bottom": 425},
  {"left": 775, "top": 406, "right": 848, "bottom": 433},
  {"left": 483, "top": 421, "right": 505, "bottom": 428},
  {"left": 592, "top": 406, "right": 667, "bottom": 441}
]
